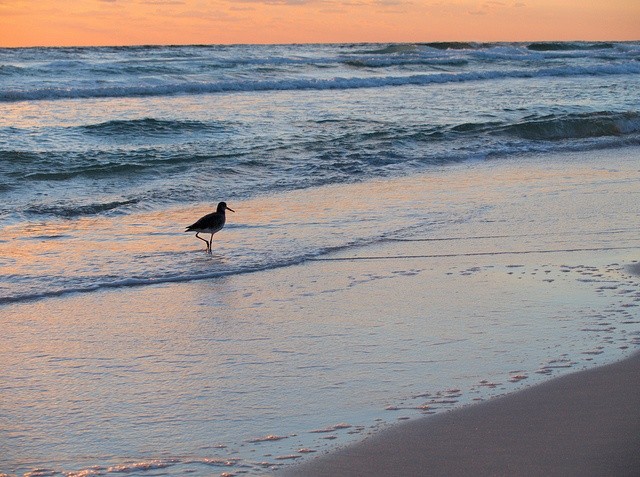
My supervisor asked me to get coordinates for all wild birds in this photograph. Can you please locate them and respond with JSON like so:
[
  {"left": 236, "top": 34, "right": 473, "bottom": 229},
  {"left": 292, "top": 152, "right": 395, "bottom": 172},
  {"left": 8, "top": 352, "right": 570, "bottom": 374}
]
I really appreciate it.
[{"left": 184, "top": 201, "right": 236, "bottom": 253}]
